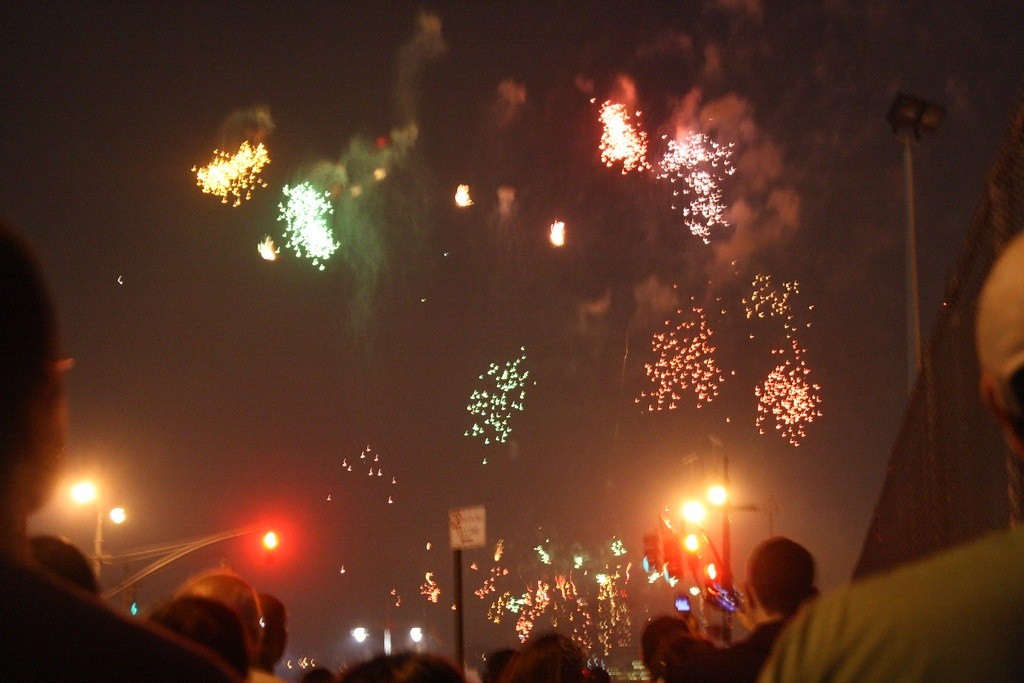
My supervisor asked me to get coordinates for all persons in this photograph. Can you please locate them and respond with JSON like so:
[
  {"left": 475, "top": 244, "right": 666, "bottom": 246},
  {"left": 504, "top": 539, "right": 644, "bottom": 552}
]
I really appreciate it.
[
  {"left": 759, "top": 233, "right": 1024, "bottom": 683},
  {"left": 480, "top": 631, "right": 611, "bottom": 683},
  {"left": 640, "top": 616, "right": 717, "bottom": 683},
  {"left": 301, "top": 651, "right": 464, "bottom": 683},
  {"left": 729, "top": 536, "right": 820, "bottom": 683},
  {"left": 0, "top": 212, "right": 241, "bottom": 683},
  {"left": 147, "top": 572, "right": 290, "bottom": 683}
]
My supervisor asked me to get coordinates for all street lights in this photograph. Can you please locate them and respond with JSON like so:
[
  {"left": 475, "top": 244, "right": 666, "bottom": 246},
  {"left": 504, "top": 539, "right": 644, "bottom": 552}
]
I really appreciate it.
[{"left": 72, "top": 482, "right": 126, "bottom": 583}]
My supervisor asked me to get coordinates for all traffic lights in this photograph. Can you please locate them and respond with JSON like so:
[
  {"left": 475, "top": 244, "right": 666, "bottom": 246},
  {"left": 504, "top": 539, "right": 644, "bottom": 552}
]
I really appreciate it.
[
  {"left": 263, "top": 521, "right": 283, "bottom": 560},
  {"left": 641, "top": 530, "right": 659, "bottom": 571}
]
